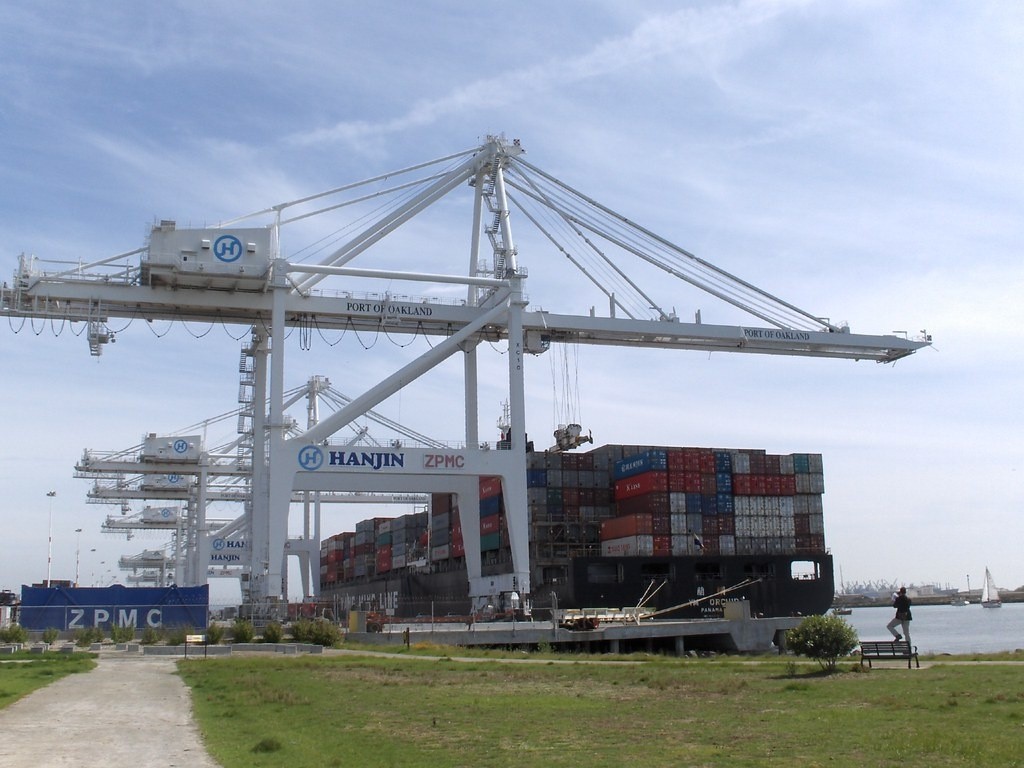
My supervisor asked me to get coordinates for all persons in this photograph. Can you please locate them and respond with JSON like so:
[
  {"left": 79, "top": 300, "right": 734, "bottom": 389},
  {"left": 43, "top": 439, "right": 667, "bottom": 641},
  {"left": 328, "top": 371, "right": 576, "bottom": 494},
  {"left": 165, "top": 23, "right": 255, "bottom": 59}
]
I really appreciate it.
[{"left": 886, "top": 586, "right": 912, "bottom": 655}]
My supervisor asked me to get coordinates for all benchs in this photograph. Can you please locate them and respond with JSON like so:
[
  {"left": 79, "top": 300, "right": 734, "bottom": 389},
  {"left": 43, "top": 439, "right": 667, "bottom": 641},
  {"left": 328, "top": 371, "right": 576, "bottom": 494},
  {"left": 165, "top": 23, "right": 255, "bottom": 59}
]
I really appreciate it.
[{"left": 859, "top": 641, "right": 920, "bottom": 669}]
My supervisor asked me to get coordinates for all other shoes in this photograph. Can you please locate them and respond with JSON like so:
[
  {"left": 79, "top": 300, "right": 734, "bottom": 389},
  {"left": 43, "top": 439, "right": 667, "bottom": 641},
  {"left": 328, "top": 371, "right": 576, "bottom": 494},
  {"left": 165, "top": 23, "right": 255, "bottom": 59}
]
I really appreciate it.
[{"left": 895, "top": 635, "right": 902, "bottom": 642}]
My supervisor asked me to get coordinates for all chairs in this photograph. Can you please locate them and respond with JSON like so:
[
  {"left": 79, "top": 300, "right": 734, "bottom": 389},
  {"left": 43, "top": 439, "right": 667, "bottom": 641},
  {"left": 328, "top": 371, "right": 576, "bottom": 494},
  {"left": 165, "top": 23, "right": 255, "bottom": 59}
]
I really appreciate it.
[{"left": 562, "top": 607, "right": 645, "bottom": 625}]
